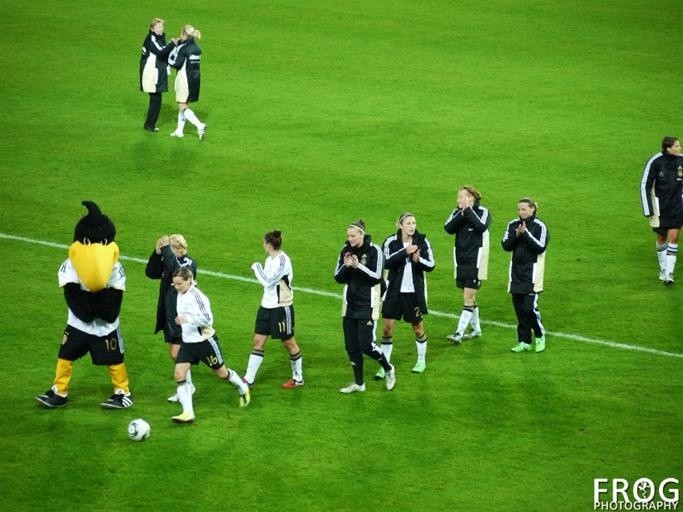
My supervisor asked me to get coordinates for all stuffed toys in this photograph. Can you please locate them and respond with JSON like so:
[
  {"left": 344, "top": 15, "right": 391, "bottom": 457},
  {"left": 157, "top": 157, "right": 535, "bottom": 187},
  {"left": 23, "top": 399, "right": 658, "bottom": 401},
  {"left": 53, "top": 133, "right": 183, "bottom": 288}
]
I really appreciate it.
[{"left": 35, "top": 201, "right": 133, "bottom": 411}]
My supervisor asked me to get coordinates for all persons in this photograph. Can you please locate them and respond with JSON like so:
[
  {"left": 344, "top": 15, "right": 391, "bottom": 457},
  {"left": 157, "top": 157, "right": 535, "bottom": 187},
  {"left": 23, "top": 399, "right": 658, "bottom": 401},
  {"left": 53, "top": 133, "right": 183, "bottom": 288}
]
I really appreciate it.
[
  {"left": 140, "top": 19, "right": 182, "bottom": 133},
  {"left": 243, "top": 229, "right": 305, "bottom": 389},
  {"left": 171, "top": 266, "right": 251, "bottom": 423},
  {"left": 374, "top": 212, "right": 436, "bottom": 380},
  {"left": 334, "top": 220, "right": 396, "bottom": 394},
  {"left": 167, "top": 24, "right": 208, "bottom": 139},
  {"left": 640, "top": 137, "right": 683, "bottom": 285},
  {"left": 501, "top": 199, "right": 549, "bottom": 353},
  {"left": 146, "top": 233, "right": 197, "bottom": 404},
  {"left": 443, "top": 184, "right": 492, "bottom": 344}
]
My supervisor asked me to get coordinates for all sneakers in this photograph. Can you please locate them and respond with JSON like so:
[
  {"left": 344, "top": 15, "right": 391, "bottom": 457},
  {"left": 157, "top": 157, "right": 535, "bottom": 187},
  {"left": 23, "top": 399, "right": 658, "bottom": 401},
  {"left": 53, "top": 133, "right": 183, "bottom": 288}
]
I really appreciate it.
[
  {"left": 384, "top": 362, "right": 396, "bottom": 391},
  {"left": 410, "top": 362, "right": 426, "bottom": 373},
  {"left": 169, "top": 128, "right": 185, "bottom": 138},
  {"left": 166, "top": 384, "right": 196, "bottom": 403},
  {"left": 239, "top": 383, "right": 250, "bottom": 408},
  {"left": 240, "top": 376, "right": 255, "bottom": 388},
  {"left": 281, "top": 378, "right": 305, "bottom": 389},
  {"left": 445, "top": 331, "right": 463, "bottom": 344},
  {"left": 143, "top": 122, "right": 159, "bottom": 133},
  {"left": 659, "top": 270, "right": 665, "bottom": 281},
  {"left": 339, "top": 381, "right": 366, "bottom": 394},
  {"left": 463, "top": 330, "right": 482, "bottom": 340},
  {"left": 510, "top": 341, "right": 532, "bottom": 353},
  {"left": 535, "top": 334, "right": 546, "bottom": 352},
  {"left": 198, "top": 123, "right": 207, "bottom": 141},
  {"left": 375, "top": 365, "right": 386, "bottom": 379},
  {"left": 664, "top": 275, "right": 674, "bottom": 286},
  {"left": 170, "top": 411, "right": 196, "bottom": 424}
]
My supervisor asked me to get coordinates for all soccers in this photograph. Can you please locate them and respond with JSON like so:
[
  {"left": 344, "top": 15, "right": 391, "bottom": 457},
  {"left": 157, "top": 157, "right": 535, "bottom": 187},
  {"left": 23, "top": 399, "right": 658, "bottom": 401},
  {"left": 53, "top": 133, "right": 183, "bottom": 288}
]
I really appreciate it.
[{"left": 128, "top": 418, "right": 151, "bottom": 441}]
